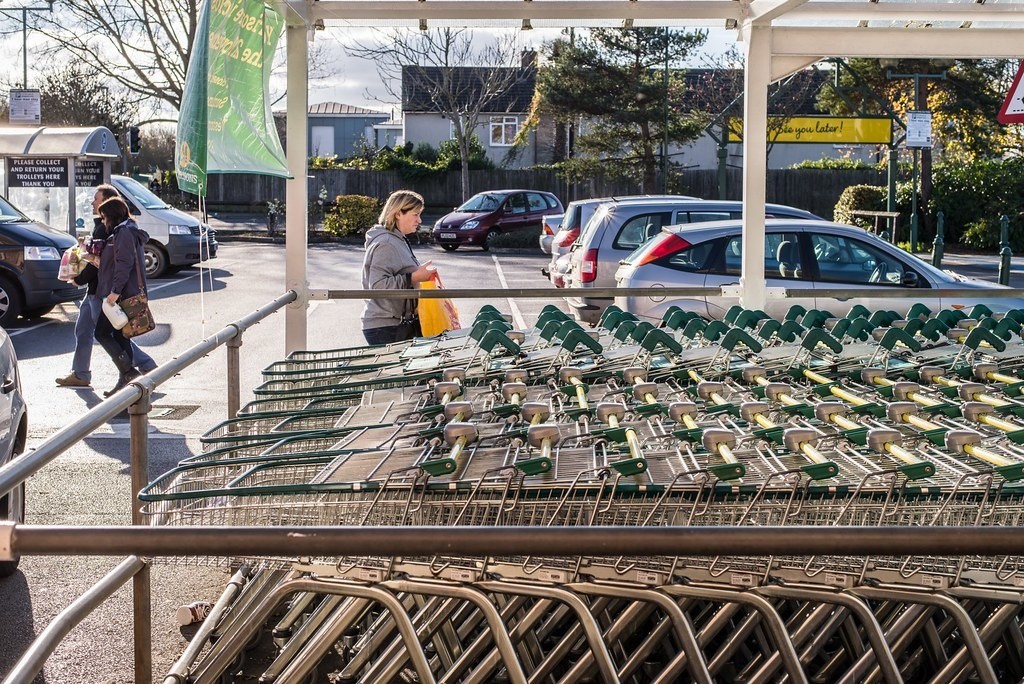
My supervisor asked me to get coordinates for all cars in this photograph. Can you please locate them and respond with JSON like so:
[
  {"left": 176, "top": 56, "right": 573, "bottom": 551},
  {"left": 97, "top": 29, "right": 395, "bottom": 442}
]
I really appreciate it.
[
  {"left": 613, "top": 218, "right": 1024, "bottom": 331},
  {"left": 433, "top": 190, "right": 567, "bottom": 253},
  {"left": 0, "top": 323, "right": 29, "bottom": 578},
  {"left": 539, "top": 195, "right": 829, "bottom": 329}
]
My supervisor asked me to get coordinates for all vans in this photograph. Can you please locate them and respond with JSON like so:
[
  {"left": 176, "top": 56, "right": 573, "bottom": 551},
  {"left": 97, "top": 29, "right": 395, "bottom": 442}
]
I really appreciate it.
[
  {"left": 1, "top": 194, "right": 92, "bottom": 328},
  {"left": 111, "top": 173, "right": 219, "bottom": 280}
]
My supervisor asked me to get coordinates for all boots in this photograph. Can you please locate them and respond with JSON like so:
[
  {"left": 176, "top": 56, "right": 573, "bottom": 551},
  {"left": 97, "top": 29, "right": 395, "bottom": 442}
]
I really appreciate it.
[{"left": 103, "top": 351, "right": 141, "bottom": 397}]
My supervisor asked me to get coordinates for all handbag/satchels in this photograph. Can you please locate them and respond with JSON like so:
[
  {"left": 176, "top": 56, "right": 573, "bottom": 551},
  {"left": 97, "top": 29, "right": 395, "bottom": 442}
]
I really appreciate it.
[
  {"left": 418, "top": 271, "right": 451, "bottom": 338},
  {"left": 434, "top": 271, "right": 460, "bottom": 330},
  {"left": 396, "top": 272, "right": 442, "bottom": 342},
  {"left": 114, "top": 291, "right": 155, "bottom": 338},
  {"left": 61, "top": 238, "right": 101, "bottom": 287}
]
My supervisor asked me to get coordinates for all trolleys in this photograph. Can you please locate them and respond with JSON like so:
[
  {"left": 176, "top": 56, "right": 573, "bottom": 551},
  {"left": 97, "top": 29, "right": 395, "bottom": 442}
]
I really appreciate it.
[{"left": 133, "top": 304, "right": 1024, "bottom": 684}]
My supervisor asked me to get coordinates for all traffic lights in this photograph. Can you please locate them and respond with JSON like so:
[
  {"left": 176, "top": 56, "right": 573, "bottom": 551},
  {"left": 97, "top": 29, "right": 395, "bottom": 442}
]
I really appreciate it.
[{"left": 129, "top": 125, "right": 141, "bottom": 154}]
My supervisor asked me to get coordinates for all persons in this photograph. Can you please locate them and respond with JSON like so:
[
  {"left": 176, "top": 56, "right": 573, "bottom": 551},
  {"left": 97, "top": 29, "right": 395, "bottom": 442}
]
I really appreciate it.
[
  {"left": 54, "top": 183, "right": 159, "bottom": 386},
  {"left": 361, "top": 189, "right": 439, "bottom": 345},
  {"left": 80, "top": 198, "right": 149, "bottom": 396}
]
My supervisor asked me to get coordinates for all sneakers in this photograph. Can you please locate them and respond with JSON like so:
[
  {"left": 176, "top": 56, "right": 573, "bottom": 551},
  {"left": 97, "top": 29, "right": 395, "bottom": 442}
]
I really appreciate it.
[{"left": 56, "top": 373, "right": 90, "bottom": 387}]
